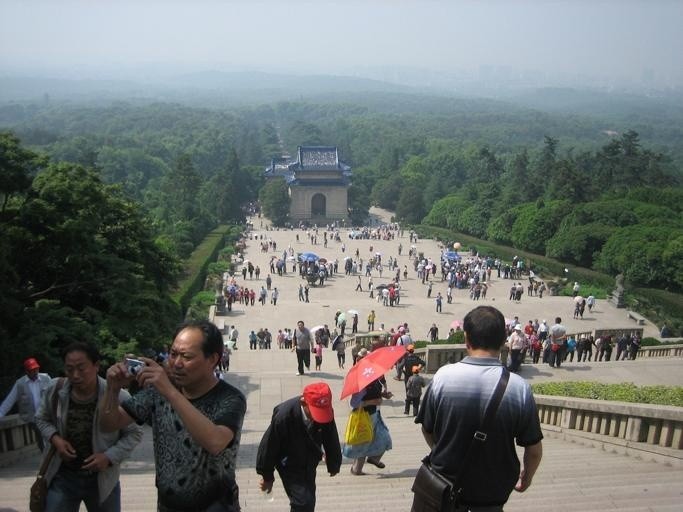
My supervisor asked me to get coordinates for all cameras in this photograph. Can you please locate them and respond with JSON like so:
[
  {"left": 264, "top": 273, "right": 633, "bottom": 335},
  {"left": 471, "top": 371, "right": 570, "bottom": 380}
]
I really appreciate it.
[{"left": 125, "top": 358, "right": 145, "bottom": 377}]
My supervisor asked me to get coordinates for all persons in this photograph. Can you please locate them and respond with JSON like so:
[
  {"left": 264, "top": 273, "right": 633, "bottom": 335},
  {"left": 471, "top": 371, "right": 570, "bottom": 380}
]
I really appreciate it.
[
  {"left": 145, "top": 348, "right": 166, "bottom": 366},
  {"left": 342, "top": 375, "right": 392, "bottom": 475},
  {"left": 0, "top": 358, "right": 52, "bottom": 454},
  {"left": 30, "top": 341, "right": 144, "bottom": 512},
  {"left": 414, "top": 306, "right": 544, "bottom": 512},
  {"left": 158, "top": 344, "right": 169, "bottom": 366},
  {"left": 100, "top": 320, "right": 246, "bottom": 512},
  {"left": 256, "top": 382, "right": 342, "bottom": 512},
  {"left": 216, "top": 207, "right": 642, "bottom": 419}
]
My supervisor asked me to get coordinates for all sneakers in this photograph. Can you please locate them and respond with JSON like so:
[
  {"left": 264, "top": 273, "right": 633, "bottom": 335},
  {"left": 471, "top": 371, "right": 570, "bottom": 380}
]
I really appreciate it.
[
  {"left": 350, "top": 466, "right": 364, "bottom": 475},
  {"left": 295, "top": 366, "right": 310, "bottom": 376},
  {"left": 221, "top": 366, "right": 229, "bottom": 373},
  {"left": 339, "top": 364, "right": 345, "bottom": 370},
  {"left": 392, "top": 376, "right": 401, "bottom": 381},
  {"left": 366, "top": 457, "right": 385, "bottom": 468}
]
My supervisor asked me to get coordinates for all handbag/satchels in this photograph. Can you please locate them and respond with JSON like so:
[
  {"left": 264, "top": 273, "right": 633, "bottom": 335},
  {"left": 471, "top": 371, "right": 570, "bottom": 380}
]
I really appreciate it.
[
  {"left": 550, "top": 344, "right": 559, "bottom": 352},
  {"left": 409, "top": 456, "right": 462, "bottom": 511},
  {"left": 345, "top": 404, "right": 374, "bottom": 445},
  {"left": 28, "top": 472, "right": 47, "bottom": 511},
  {"left": 293, "top": 329, "right": 297, "bottom": 346}
]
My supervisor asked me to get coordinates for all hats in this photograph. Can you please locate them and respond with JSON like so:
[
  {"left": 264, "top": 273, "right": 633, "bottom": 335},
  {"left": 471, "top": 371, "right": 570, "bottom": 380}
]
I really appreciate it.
[
  {"left": 580, "top": 333, "right": 586, "bottom": 340},
  {"left": 407, "top": 344, "right": 413, "bottom": 352},
  {"left": 304, "top": 382, "right": 335, "bottom": 424},
  {"left": 411, "top": 365, "right": 419, "bottom": 373},
  {"left": 358, "top": 348, "right": 370, "bottom": 356},
  {"left": 513, "top": 323, "right": 522, "bottom": 331},
  {"left": 398, "top": 326, "right": 405, "bottom": 333},
  {"left": 24, "top": 358, "right": 40, "bottom": 371}
]
